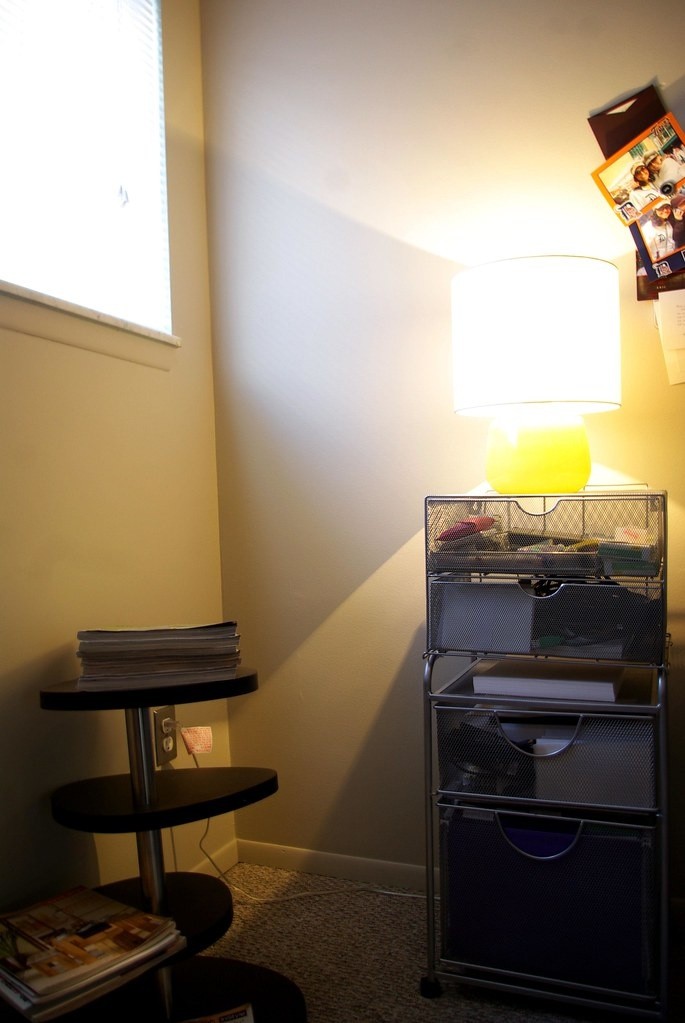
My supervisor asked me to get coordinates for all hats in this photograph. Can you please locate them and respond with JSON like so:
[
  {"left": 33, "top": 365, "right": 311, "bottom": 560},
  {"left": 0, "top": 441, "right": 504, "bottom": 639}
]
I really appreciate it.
[
  {"left": 670, "top": 194, "right": 685, "bottom": 208},
  {"left": 631, "top": 161, "right": 645, "bottom": 175},
  {"left": 653, "top": 199, "right": 671, "bottom": 212},
  {"left": 642, "top": 150, "right": 659, "bottom": 167}
]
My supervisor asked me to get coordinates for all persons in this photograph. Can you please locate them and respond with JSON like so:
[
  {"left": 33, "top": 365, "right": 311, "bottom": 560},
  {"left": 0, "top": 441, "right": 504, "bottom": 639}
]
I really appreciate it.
[
  {"left": 629, "top": 149, "right": 684, "bottom": 210},
  {"left": 640, "top": 194, "right": 685, "bottom": 260}
]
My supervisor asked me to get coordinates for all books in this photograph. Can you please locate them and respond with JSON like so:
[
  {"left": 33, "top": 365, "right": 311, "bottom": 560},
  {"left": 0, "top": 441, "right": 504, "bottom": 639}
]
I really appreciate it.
[
  {"left": 76, "top": 620, "right": 243, "bottom": 691},
  {"left": 0, "top": 884, "right": 187, "bottom": 1022},
  {"left": 472, "top": 660, "right": 627, "bottom": 702}
]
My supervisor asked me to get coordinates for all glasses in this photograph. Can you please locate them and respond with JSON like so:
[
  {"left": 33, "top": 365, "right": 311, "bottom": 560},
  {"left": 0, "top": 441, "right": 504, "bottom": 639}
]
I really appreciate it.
[
  {"left": 656, "top": 205, "right": 669, "bottom": 211},
  {"left": 635, "top": 166, "right": 646, "bottom": 174},
  {"left": 648, "top": 157, "right": 658, "bottom": 167}
]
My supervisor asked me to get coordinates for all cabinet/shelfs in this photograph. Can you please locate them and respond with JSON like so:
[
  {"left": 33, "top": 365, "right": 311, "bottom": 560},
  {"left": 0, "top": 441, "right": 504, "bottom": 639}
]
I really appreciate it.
[
  {"left": 40, "top": 667, "right": 308, "bottom": 1023},
  {"left": 419, "top": 496, "right": 685, "bottom": 1022}
]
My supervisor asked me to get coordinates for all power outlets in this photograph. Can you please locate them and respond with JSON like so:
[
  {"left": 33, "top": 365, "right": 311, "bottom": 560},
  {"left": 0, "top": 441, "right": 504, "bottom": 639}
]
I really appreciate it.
[{"left": 154, "top": 706, "right": 178, "bottom": 767}]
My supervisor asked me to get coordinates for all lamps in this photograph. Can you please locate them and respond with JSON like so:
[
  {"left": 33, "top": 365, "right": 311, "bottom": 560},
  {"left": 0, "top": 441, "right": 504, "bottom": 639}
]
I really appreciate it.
[{"left": 449, "top": 253, "right": 622, "bottom": 495}]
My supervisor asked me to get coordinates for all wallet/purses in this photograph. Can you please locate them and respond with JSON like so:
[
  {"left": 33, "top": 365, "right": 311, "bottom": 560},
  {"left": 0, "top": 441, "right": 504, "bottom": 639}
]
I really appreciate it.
[{"left": 436, "top": 516, "right": 495, "bottom": 541}]
